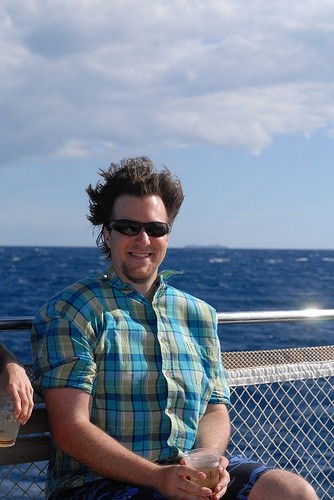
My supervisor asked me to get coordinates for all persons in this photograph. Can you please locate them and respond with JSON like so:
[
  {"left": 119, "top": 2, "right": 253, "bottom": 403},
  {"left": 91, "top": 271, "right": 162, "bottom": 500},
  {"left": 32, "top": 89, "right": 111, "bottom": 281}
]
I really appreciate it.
[
  {"left": 0, "top": 341, "right": 35, "bottom": 425},
  {"left": 32, "top": 155, "right": 322, "bottom": 500}
]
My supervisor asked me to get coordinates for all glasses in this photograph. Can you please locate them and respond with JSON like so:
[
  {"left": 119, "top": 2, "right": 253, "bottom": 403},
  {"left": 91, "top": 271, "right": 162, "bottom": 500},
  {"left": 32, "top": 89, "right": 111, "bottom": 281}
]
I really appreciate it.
[{"left": 106, "top": 219, "right": 171, "bottom": 237}]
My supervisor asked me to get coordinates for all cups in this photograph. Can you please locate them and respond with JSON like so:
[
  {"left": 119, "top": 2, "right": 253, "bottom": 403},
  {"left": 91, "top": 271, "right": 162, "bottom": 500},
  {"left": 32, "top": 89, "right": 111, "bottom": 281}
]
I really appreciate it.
[
  {"left": 1, "top": 394, "right": 20, "bottom": 448},
  {"left": 183, "top": 448, "right": 220, "bottom": 500}
]
